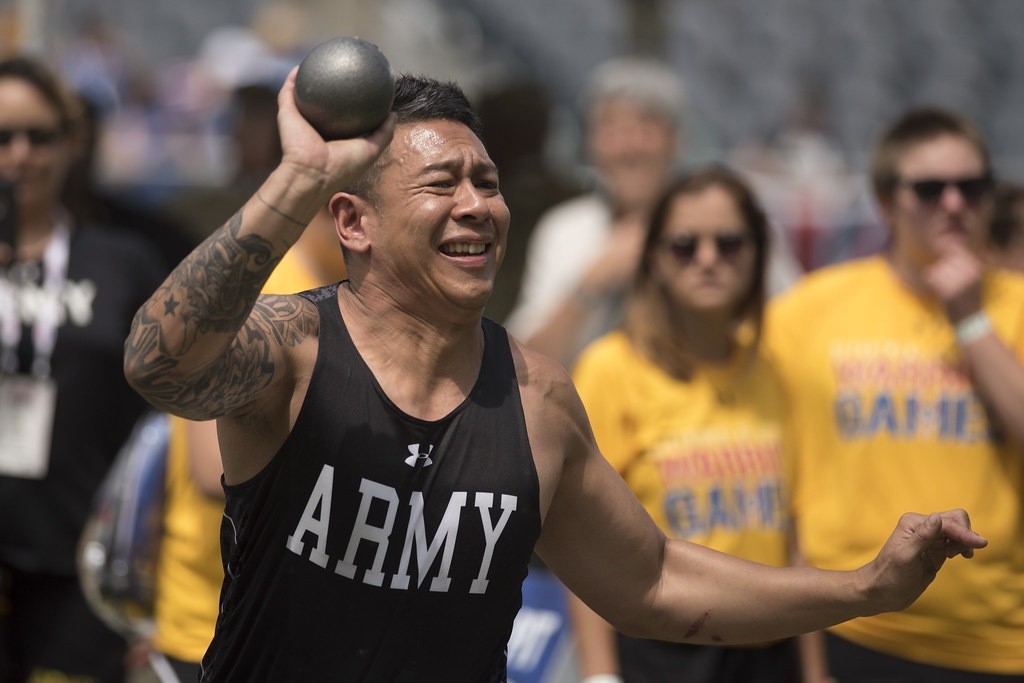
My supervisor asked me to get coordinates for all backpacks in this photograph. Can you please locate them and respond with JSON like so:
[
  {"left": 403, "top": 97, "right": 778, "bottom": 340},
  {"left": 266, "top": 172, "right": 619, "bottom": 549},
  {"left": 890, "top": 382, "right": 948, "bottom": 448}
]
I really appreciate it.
[{"left": 75, "top": 411, "right": 169, "bottom": 644}]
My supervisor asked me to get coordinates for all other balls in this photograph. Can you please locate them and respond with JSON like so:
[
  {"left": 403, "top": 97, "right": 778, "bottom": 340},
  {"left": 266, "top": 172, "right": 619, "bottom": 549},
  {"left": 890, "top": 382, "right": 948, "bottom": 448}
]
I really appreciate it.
[{"left": 291, "top": 33, "right": 400, "bottom": 144}]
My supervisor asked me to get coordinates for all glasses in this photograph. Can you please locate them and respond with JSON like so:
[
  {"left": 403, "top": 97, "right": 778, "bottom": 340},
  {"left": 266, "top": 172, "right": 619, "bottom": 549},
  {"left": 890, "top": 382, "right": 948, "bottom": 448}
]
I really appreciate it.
[
  {"left": 898, "top": 169, "right": 991, "bottom": 205},
  {"left": 0, "top": 127, "right": 64, "bottom": 146},
  {"left": 661, "top": 228, "right": 752, "bottom": 258}
]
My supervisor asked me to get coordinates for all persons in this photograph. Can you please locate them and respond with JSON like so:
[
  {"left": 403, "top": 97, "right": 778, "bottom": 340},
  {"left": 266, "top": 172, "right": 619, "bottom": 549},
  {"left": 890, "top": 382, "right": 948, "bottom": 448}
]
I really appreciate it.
[{"left": 0, "top": 0, "right": 1024, "bottom": 683}]
